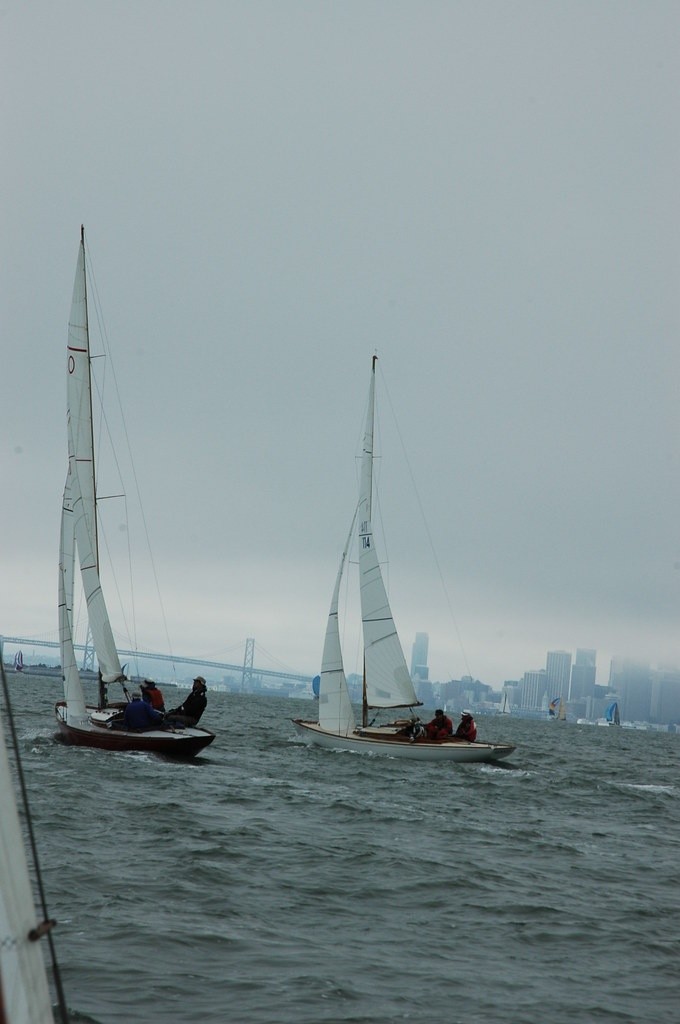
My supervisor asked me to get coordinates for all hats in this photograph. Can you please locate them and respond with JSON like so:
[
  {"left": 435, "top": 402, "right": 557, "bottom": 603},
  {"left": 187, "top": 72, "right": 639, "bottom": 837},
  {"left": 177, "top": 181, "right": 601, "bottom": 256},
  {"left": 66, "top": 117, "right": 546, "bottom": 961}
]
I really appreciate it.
[
  {"left": 461, "top": 708, "right": 470, "bottom": 716},
  {"left": 193, "top": 676, "right": 206, "bottom": 685},
  {"left": 436, "top": 710, "right": 443, "bottom": 715},
  {"left": 145, "top": 678, "right": 156, "bottom": 685},
  {"left": 131, "top": 691, "right": 141, "bottom": 700}
]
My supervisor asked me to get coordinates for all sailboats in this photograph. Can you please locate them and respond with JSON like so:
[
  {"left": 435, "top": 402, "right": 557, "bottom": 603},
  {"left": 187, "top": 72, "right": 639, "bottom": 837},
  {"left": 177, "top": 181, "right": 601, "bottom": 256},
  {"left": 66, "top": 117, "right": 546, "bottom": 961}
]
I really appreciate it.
[
  {"left": 53, "top": 219, "right": 219, "bottom": 764},
  {"left": 312, "top": 676, "right": 321, "bottom": 700},
  {"left": 496, "top": 689, "right": 512, "bottom": 718},
  {"left": 13, "top": 649, "right": 25, "bottom": 673},
  {"left": 283, "top": 353, "right": 519, "bottom": 763},
  {"left": 606, "top": 701, "right": 623, "bottom": 728},
  {"left": 0, "top": 642, "right": 97, "bottom": 1024},
  {"left": 546, "top": 694, "right": 568, "bottom": 721}
]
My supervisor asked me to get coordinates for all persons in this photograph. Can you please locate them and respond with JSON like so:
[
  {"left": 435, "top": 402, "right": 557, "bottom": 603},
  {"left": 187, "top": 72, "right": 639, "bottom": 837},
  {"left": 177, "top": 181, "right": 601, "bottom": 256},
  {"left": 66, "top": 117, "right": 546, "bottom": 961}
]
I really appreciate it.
[
  {"left": 426, "top": 709, "right": 453, "bottom": 740},
  {"left": 456, "top": 709, "right": 477, "bottom": 742},
  {"left": 160, "top": 676, "right": 208, "bottom": 730},
  {"left": 124, "top": 689, "right": 161, "bottom": 729},
  {"left": 142, "top": 678, "right": 165, "bottom": 712}
]
